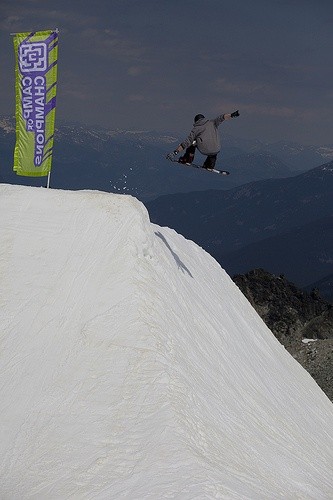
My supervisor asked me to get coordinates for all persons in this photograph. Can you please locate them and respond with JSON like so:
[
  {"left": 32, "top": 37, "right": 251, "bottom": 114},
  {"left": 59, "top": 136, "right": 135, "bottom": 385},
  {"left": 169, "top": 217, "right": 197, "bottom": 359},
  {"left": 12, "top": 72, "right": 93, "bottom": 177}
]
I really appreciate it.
[{"left": 167, "top": 110, "right": 239, "bottom": 170}]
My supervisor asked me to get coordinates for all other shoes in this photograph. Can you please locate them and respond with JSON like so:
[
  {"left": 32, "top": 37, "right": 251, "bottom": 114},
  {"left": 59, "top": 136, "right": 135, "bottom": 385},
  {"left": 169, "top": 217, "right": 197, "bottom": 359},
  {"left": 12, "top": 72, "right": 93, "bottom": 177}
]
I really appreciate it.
[
  {"left": 179, "top": 156, "right": 192, "bottom": 165},
  {"left": 202, "top": 164, "right": 214, "bottom": 170}
]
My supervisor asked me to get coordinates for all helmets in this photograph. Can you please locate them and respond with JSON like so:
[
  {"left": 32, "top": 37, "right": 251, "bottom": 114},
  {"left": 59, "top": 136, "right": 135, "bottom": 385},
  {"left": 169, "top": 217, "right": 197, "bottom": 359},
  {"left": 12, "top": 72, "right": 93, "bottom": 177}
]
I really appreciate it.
[{"left": 195, "top": 114, "right": 205, "bottom": 122}]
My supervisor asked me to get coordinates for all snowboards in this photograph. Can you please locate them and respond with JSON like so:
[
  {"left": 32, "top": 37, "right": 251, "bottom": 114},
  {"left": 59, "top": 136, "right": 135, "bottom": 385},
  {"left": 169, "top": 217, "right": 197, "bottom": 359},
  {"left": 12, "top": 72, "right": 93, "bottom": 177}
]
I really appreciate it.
[{"left": 168, "top": 158, "right": 230, "bottom": 176}]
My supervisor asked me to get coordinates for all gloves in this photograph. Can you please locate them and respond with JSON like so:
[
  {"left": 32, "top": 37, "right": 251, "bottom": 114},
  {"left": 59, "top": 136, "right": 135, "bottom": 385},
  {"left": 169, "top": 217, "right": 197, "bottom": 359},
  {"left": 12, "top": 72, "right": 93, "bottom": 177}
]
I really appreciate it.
[
  {"left": 167, "top": 152, "right": 176, "bottom": 161},
  {"left": 231, "top": 110, "right": 239, "bottom": 118}
]
